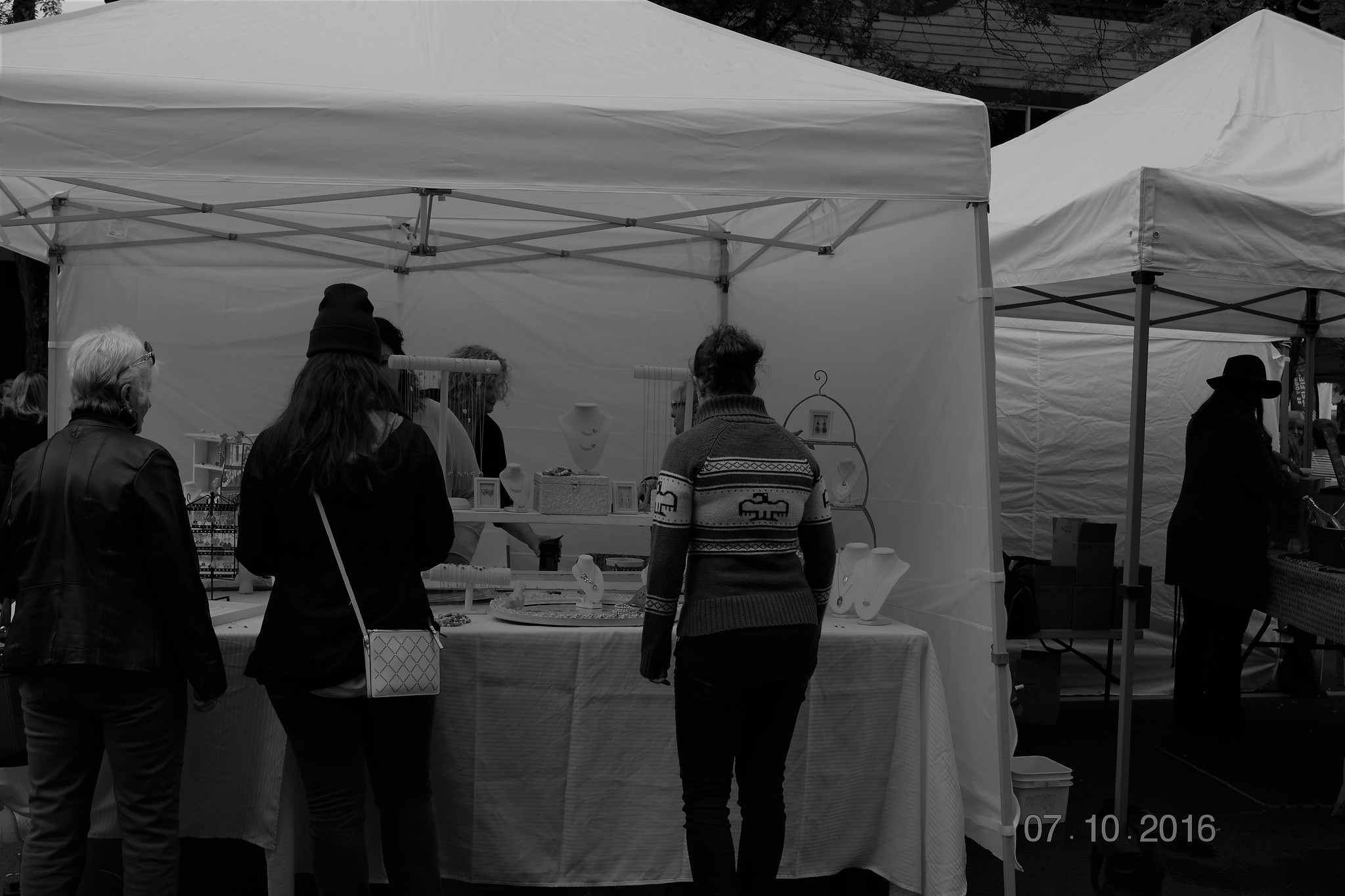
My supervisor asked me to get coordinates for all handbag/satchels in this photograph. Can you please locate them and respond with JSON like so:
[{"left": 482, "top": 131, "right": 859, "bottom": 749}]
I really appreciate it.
[
  {"left": 1002, "top": 550, "right": 1040, "bottom": 636},
  {"left": 363, "top": 626, "right": 443, "bottom": 698},
  {"left": 0, "top": 597, "right": 29, "bottom": 767}
]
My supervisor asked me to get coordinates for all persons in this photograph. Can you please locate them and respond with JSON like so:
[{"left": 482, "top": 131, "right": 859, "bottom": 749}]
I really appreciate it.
[
  {"left": 641, "top": 323, "right": 833, "bottom": 896},
  {"left": 0, "top": 271, "right": 700, "bottom": 896},
  {"left": 1161, "top": 356, "right": 1344, "bottom": 822}
]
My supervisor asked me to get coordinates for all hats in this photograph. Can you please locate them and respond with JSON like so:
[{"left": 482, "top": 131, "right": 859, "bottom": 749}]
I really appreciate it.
[
  {"left": 306, "top": 283, "right": 381, "bottom": 368},
  {"left": 1206, "top": 354, "right": 1282, "bottom": 399}
]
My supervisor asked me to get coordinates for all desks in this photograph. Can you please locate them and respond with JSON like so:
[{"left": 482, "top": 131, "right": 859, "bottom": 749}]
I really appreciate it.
[
  {"left": 1008, "top": 629, "right": 1147, "bottom": 707},
  {"left": 1240, "top": 551, "right": 1345, "bottom": 676},
  {"left": 91, "top": 595, "right": 970, "bottom": 896}
]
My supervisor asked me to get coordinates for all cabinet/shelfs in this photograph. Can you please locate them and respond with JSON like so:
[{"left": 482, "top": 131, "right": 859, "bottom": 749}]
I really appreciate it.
[
  {"left": 181, "top": 432, "right": 237, "bottom": 521},
  {"left": 782, "top": 369, "right": 878, "bottom": 551}
]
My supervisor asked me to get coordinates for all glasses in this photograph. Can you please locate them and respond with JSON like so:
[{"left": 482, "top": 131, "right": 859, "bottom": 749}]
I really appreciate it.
[
  {"left": 115, "top": 341, "right": 155, "bottom": 378},
  {"left": 671, "top": 400, "right": 699, "bottom": 411}
]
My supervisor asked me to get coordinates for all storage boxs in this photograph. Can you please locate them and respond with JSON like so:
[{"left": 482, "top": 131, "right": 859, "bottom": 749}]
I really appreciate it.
[
  {"left": 1003, "top": 515, "right": 1153, "bottom": 725},
  {"left": 1010, "top": 755, "right": 1074, "bottom": 826},
  {"left": 533, "top": 472, "right": 609, "bottom": 517},
  {"left": 1307, "top": 523, "right": 1344, "bottom": 566}
]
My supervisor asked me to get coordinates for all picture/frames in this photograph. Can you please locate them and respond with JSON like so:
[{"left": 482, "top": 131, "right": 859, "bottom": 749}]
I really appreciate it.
[
  {"left": 612, "top": 481, "right": 639, "bottom": 515},
  {"left": 474, "top": 477, "right": 500, "bottom": 512},
  {"left": 807, "top": 409, "right": 834, "bottom": 442}
]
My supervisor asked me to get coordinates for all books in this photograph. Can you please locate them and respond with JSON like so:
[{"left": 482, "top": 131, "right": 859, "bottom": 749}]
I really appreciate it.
[{"left": 0, "top": 0, "right": 1027, "bottom": 896}]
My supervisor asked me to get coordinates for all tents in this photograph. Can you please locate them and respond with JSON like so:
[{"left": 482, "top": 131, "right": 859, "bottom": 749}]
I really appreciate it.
[{"left": 987, "top": 8, "right": 1345, "bottom": 847}]
